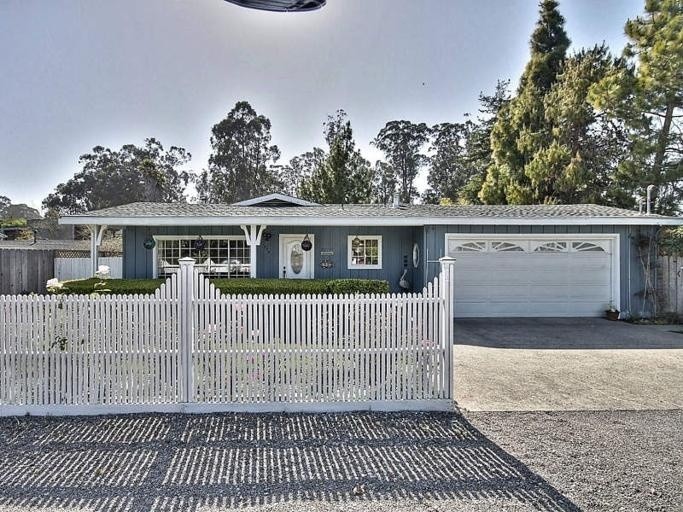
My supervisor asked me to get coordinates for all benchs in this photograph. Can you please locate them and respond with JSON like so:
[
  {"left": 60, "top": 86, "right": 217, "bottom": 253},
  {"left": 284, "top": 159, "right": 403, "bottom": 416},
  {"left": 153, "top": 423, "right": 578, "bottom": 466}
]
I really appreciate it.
[{"left": 163, "top": 263, "right": 250, "bottom": 279}]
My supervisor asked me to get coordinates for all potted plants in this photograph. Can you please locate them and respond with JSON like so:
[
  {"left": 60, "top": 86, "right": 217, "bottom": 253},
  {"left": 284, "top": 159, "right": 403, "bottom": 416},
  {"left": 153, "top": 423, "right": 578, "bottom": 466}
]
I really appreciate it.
[{"left": 606, "top": 297, "right": 620, "bottom": 321}]
